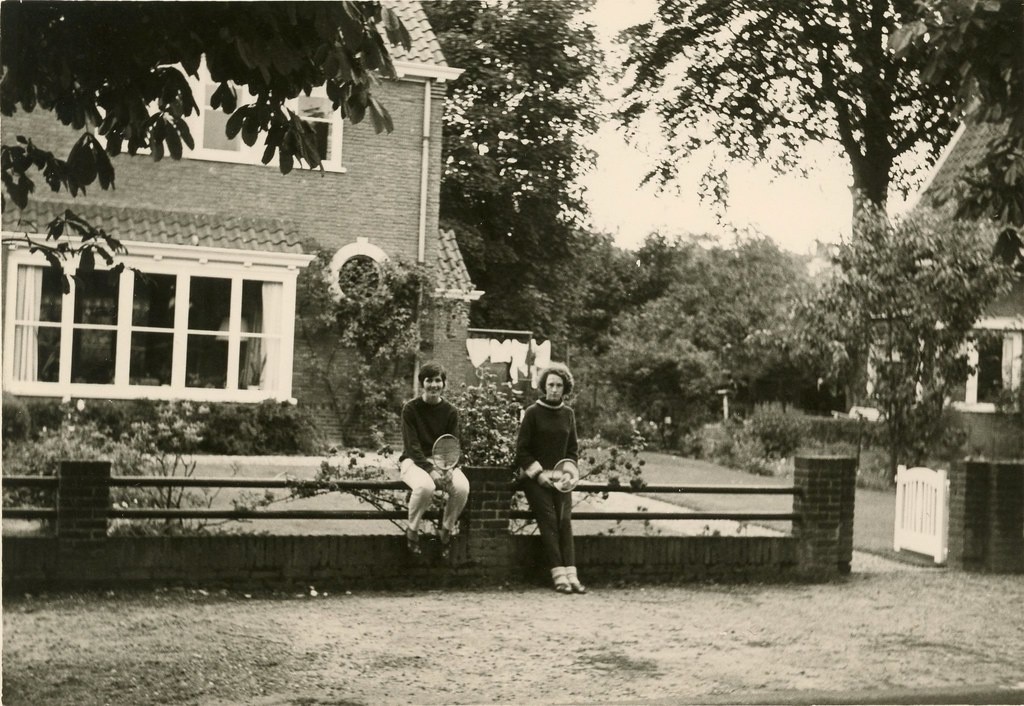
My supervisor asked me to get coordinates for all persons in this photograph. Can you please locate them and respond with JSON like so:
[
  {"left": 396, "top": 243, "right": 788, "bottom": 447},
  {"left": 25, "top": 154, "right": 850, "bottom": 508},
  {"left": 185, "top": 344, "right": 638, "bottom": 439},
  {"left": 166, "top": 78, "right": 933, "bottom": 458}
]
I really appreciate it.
[
  {"left": 516, "top": 366, "right": 587, "bottom": 593},
  {"left": 398, "top": 361, "right": 471, "bottom": 559}
]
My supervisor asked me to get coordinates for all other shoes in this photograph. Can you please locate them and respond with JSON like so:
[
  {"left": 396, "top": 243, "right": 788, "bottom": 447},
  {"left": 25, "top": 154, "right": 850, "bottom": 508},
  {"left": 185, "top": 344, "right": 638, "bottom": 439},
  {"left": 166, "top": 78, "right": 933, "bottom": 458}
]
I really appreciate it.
[
  {"left": 439, "top": 536, "right": 457, "bottom": 558},
  {"left": 553, "top": 577, "right": 573, "bottom": 594},
  {"left": 569, "top": 574, "right": 589, "bottom": 593},
  {"left": 406, "top": 528, "right": 423, "bottom": 556}
]
